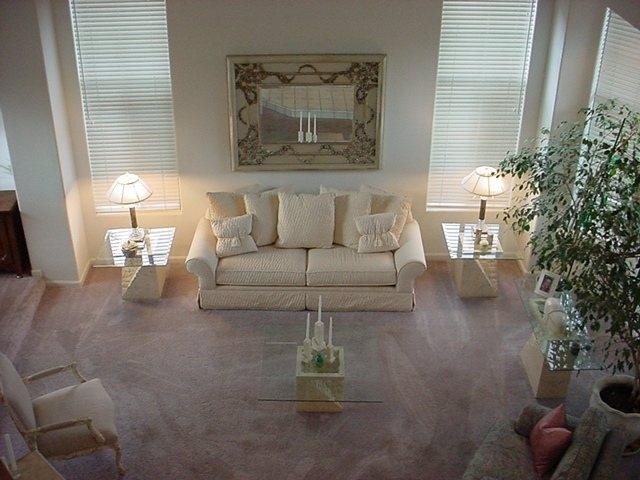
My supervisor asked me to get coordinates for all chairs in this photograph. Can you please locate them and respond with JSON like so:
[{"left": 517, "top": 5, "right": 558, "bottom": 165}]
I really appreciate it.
[
  {"left": 0, "top": 352, "right": 126, "bottom": 480},
  {"left": 463, "top": 402, "right": 629, "bottom": 479}
]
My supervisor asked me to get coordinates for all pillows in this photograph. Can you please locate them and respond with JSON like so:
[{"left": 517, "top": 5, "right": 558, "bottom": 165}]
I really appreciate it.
[
  {"left": 203, "top": 184, "right": 411, "bottom": 259},
  {"left": 527, "top": 403, "right": 574, "bottom": 480}
]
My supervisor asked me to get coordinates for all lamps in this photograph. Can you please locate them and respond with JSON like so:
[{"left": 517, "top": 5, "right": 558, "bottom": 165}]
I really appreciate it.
[
  {"left": 105, "top": 172, "right": 152, "bottom": 243},
  {"left": 461, "top": 165, "right": 507, "bottom": 234}
]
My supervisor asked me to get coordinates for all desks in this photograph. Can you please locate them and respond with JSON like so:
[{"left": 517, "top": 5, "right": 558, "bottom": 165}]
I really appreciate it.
[
  {"left": 514, "top": 275, "right": 607, "bottom": 401},
  {"left": 440, "top": 222, "right": 524, "bottom": 299},
  {"left": 91, "top": 227, "right": 176, "bottom": 301}
]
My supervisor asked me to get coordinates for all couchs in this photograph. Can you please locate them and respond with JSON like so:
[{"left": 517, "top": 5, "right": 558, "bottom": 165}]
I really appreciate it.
[{"left": 184, "top": 184, "right": 429, "bottom": 314}]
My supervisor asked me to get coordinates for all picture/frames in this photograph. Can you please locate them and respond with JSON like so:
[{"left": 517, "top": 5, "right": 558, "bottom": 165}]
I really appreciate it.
[
  {"left": 534, "top": 268, "right": 561, "bottom": 299},
  {"left": 226, "top": 53, "right": 389, "bottom": 171}
]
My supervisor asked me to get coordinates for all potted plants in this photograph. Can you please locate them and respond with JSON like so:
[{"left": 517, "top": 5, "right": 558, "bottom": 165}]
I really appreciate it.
[{"left": 488, "top": 99, "right": 640, "bottom": 480}]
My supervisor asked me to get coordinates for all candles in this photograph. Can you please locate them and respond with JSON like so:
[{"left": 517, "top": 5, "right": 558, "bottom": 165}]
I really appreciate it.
[{"left": 303, "top": 294, "right": 335, "bottom": 346}]
